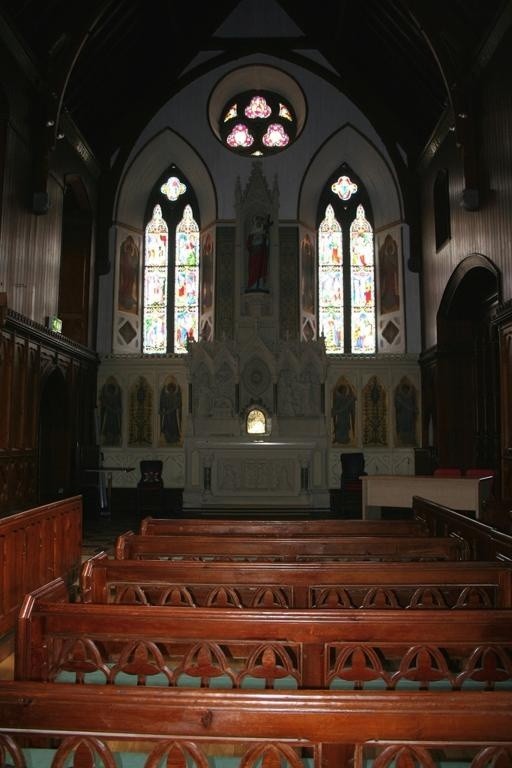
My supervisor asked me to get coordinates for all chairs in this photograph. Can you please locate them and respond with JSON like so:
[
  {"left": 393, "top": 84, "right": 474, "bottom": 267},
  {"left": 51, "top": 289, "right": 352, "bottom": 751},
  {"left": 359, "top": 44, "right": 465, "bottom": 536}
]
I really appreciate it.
[
  {"left": 432, "top": 468, "right": 496, "bottom": 503},
  {"left": 137, "top": 460, "right": 164, "bottom": 507},
  {"left": 340, "top": 452, "right": 368, "bottom": 493}
]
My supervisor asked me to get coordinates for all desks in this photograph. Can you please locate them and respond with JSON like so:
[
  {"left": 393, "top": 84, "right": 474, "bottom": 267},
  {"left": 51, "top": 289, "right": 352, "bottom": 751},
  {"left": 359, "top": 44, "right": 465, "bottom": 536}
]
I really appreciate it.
[
  {"left": 358, "top": 473, "right": 496, "bottom": 521},
  {"left": 82, "top": 465, "right": 136, "bottom": 515}
]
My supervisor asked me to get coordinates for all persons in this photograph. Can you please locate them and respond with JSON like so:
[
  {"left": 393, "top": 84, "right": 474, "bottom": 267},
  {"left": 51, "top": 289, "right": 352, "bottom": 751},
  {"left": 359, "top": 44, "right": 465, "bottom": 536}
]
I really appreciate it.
[
  {"left": 200, "top": 240, "right": 213, "bottom": 308},
  {"left": 158, "top": 381, "right": 182, "bottom": 444},
  {"left": 118, "top": 241, "right": 140, "bottom": 312},
  {"left": 378, "top": 238, "right": 398, "bottom": 311},
  {"left": 244, "top": 215, "right": 270, "bottom": 291},
  {"left": 330, "top": 382, "right": 356, "bottom": 444},
  {"left": 300, "top": 238, "right": 314, "bottom": 312},
  {"left": 392, "top": 380, "right": 418, "bottom": 446},
  {"left": 98, "top": 382, "right": 123, "bottom": 446}
]
